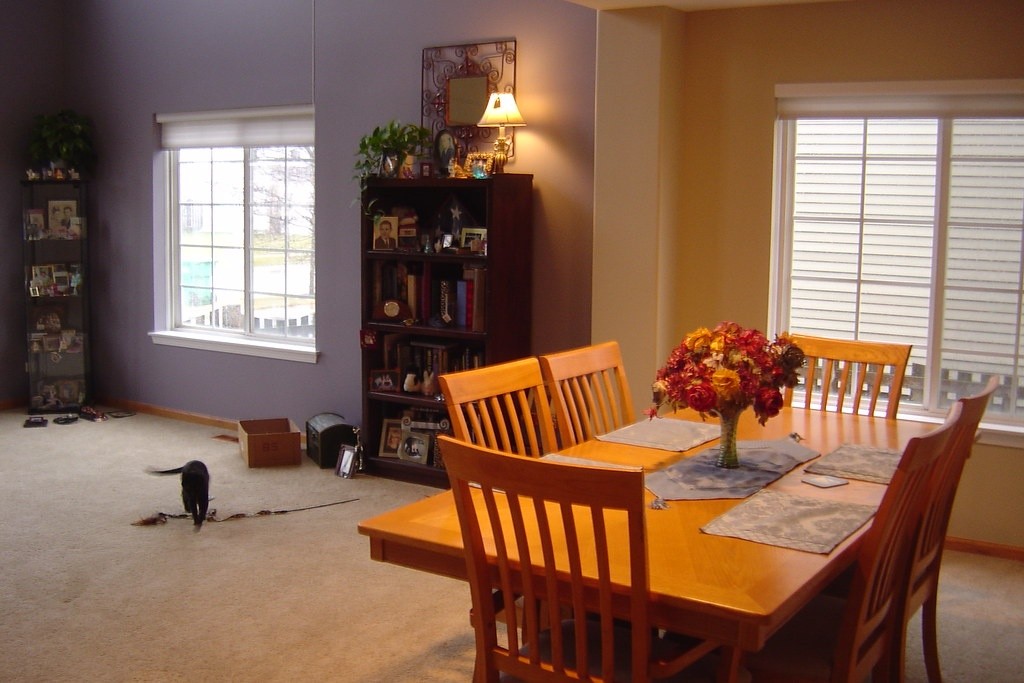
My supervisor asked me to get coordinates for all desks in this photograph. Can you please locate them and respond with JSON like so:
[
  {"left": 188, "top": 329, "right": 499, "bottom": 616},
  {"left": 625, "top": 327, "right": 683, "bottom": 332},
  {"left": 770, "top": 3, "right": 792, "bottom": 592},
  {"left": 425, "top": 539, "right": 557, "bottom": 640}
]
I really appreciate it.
[{"left": 357, "top": 404, "right": 983, "bottom": 683}]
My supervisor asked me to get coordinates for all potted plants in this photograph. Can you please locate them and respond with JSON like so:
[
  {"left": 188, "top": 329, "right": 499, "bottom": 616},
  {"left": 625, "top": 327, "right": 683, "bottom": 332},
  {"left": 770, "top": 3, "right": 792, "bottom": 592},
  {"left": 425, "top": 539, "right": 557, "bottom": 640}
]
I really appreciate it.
[{"left": 353, "top": 119, "right": 434, "bottom": 227}]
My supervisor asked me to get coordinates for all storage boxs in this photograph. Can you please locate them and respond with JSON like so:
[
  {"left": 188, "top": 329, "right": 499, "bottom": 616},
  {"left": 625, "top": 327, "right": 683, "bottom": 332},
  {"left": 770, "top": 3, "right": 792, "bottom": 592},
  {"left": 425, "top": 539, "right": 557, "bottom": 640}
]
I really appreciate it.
[
  {"left": 239, "top": 417, "right": 302, "bottom": 468},
  {"left": 305, "top": 412, "right": 358, "bottom": 469}
]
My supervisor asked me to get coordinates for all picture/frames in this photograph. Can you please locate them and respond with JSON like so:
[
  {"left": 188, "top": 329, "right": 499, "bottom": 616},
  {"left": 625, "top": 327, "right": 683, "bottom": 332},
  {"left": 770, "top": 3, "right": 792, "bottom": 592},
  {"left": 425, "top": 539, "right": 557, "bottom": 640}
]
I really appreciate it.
[
  {"left": 334, "top": 445, "right": 357, "bottom": 478},
  {"left": 373, "top": 216, "right": 399, "bottom": 251},
  {"left": 419, "top": 129, "right": 497, "bottom": 177},
  {"left": 460, "top": 228, "right": 487, "bottom": 248},
  {"left": 29, "top": 330, "right": 78, "bottom": 352},
  {"left": 32, "top": 264, "right": 71, "bottom": 295},
  {"left": 59, "top": 380, "right": 81, "bottom": 403},
  {"left": 379, "top": 417, "right": 449, "bottom": 469},
  {"left": 25, "top": 200, "right": 81, "bottom": 238},
  {"left": 442, "top": 234, "right": 453, "bottom": 249},
  {"left": 370, "top": 367, "right": 400, "bottom": 392}
]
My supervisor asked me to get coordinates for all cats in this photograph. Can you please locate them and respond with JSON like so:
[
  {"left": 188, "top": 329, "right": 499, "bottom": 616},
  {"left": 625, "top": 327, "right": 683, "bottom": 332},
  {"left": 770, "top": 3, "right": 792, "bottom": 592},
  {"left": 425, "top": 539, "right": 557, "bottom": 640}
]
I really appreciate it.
[{"left": 152, "top": 460, "right": 209, "bottom": 532}]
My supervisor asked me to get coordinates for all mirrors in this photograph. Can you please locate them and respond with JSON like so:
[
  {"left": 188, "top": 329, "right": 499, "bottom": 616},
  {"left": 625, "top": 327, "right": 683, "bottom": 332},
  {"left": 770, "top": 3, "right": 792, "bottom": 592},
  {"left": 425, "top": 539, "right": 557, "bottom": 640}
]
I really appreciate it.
[{"left": 419, "top": 39, "right": 515, "bottom": 161}]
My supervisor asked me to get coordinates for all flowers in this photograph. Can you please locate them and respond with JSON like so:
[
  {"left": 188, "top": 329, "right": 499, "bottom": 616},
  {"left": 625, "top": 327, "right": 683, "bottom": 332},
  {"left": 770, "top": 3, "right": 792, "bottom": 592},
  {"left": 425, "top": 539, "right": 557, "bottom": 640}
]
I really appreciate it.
[{"left": 642, "top": 321, "right": 808, "bottom": 459}]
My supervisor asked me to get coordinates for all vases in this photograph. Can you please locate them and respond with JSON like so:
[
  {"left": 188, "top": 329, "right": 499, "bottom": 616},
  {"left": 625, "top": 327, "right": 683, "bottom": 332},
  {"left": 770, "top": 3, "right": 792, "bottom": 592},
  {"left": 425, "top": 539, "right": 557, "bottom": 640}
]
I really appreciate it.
[{"left": 714, "top": 408, "right": 739, "bottom": 469}]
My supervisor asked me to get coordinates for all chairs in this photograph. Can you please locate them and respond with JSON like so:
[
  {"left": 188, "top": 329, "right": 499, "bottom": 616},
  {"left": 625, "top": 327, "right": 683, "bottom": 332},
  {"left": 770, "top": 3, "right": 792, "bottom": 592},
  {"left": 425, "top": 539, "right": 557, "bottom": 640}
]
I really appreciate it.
[
  {"left": 720, "top": 374, "right": 1000, "bottom": 683},
  {"left": 438, "top": 356, "right": 558, "bottom": 466},
  {"left": 438, "top": 431, "right": 722, "bottom": 683},
  {"left": 538, "top": 340, "right": 635, "bottom": 450},
  {"left": 783, "top": 334, "right": 914, "bottom": 419}
]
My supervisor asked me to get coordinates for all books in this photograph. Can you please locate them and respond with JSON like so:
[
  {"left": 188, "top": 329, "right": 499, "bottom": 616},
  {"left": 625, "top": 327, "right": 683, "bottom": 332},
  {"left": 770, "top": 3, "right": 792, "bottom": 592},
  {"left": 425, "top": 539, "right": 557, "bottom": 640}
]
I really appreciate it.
[{"left": 365, "top": 258, "right": 486, "bottom": 401}]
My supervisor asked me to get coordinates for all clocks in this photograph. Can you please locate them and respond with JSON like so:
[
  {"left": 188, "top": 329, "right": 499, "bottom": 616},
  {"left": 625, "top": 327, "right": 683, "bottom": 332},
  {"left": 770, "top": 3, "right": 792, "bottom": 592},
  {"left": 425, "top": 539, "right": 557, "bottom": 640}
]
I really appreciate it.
[{"left": 372, "top": 300, "right": 411, "bottom": 322}]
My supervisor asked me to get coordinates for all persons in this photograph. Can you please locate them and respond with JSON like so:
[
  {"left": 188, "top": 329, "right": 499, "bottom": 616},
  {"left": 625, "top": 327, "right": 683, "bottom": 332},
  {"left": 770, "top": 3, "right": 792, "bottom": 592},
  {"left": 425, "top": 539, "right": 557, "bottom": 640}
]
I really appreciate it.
[
  {"left": 60, "top": 207, "right": 73, "bottom": 229},
  {"left": 374, "top": 221, "right": 397, "bottom": 250},
  {"left": 48, "top": 206, "right": 62, "bottom": 229}
]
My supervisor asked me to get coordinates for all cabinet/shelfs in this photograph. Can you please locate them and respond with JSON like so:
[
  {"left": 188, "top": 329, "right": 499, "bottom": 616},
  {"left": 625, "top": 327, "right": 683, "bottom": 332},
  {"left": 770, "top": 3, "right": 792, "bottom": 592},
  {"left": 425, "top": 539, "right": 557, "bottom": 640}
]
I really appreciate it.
[
  {"left": 21, "top": 176, "right": 95, "bottom": 416},
  {"left": 360, "top": 174, "right": 535, "bottom": 487}
]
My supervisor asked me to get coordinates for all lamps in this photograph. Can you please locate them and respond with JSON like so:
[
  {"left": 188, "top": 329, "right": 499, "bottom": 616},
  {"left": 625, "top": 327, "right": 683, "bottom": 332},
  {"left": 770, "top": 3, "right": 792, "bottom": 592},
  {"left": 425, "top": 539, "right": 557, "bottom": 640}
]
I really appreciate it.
[{"left": 477, "top": 90, "right": 526, "bottom": 171}]
